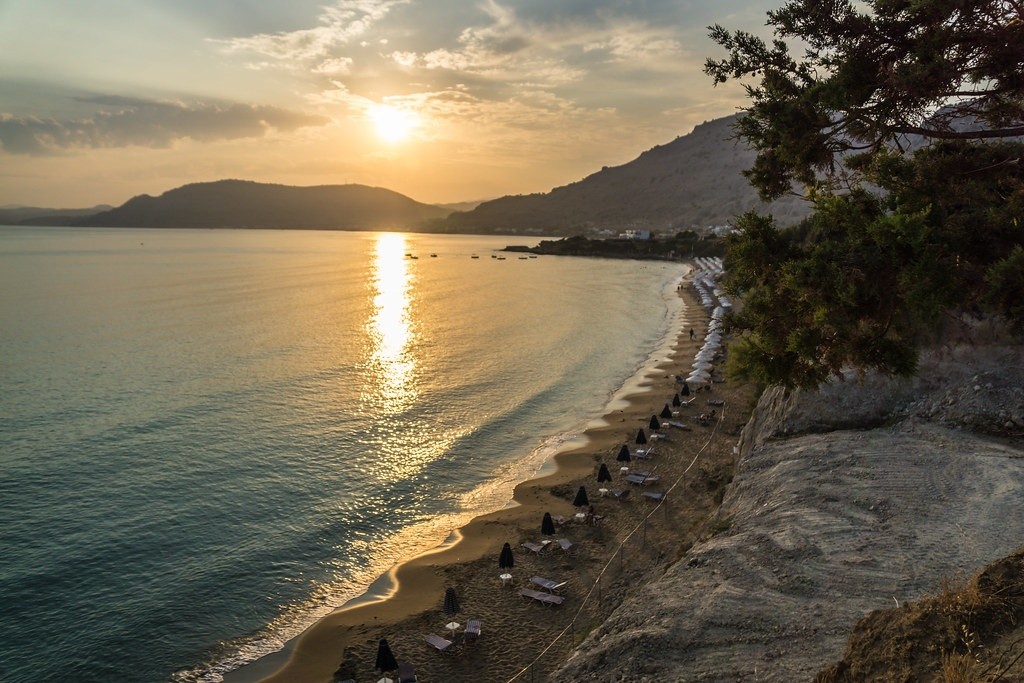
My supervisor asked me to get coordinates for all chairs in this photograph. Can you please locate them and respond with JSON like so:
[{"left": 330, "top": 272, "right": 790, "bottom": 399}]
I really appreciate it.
[
  {"left": 397, "top": 663, "right": 417, "bottom": 683},
  {"left": 629, "top": 464, "right": 658, "bottom": 477},
  {"left": 630, "top": 443, "right": 656, "bottom": 461},
  {"left": 463, "top": 618, "right": 483, "bottom": 642},
  {"left": 626, "top": 474, "right": 662, "bottom": 492},
  {"left": 649, "top": 327, "right": 726, "bottom": 441},
  {"left": 556, "top": 538, "right": 580, "bottom": 559},
  {"left": 521, "top": 588, "right": 565, "bottom": 608},
  {"left": 551, "top": 514, "right": 576, "bottom": 536},
  {"left": 521, "top": 541, "right": 552, "bottom": 559},
  {"left": 530, "top": 575, "right": 568, "bottom": 596},
  {"left": 610, "top": 488, "right": 631, "bottom": 502},
  {"left": 424, "top": 633, "right": 458, "bottom": 659}
]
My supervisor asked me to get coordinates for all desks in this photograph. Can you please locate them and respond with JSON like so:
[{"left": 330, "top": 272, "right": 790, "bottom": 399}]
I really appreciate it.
[
  {"left": 619, "top": 467, "right": 629, "bottom": 476},
  {"left": 446, "top": 622, "right": 461, "bottom": 637},
  {"left": 377, "top": 677, "right": 393, "bottom": 683},
  {"left": 500, "top": 574, "right": 514, "bottom": 587},
  {"left": 599, "top": 488, "right": 608, "bottom": 497},
  {"left": 576, "top": 513, "right": 586, "bottom": 523}
]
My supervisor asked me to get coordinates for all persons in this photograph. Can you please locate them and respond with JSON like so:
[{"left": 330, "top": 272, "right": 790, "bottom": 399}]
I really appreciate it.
[
  {"left": 709, "top": 409, "right": 715, "bottom": 417},
  {"left": 678, "top": 285, "right": 683, "bottom": 291},
  {"left": 690, "top": 328, "right": 693, "bottom": 339}
]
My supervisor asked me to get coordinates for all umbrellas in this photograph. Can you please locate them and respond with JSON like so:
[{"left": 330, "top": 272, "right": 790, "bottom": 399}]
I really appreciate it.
[
  {"left": 616, "top": 445, "right": 631, "bottom": 467},
  {"left": 680, "top": 384, "right": 690, "bottom": 402},
  {"left": 660, "top": 405, "right": 672, "bottom": 423},
  {"left": 685, "top": 333, "right": 722, "bottom": 383},
  {"left": 573, "top": 486, "right": 589, "bottom": 515},
  {"left": 597, "top": 463, "right": 613, "bottom": 489},
  {"left": 541, "top": 512, "right": 555, "bottom": 541},
  {"left": 636, "top": 429, "right": 647, "bottom": 450},
  {"left": 673, "top": 394, "right": 681, "bottom": 412},
  {"left": 499, "top": 543, "right": 513, "bottom": 577},
  {"left": 375, "top": 639, "right": 399, "bottom": 683},
  {"left": 692, "top": 257, "right": 732, "bottom": 332},
  {"left": 649, "top": 415, "right": 660, "bottom": 434},
  {"left": 444, "top": 587, "right": 460, "bottom": 625}
]
